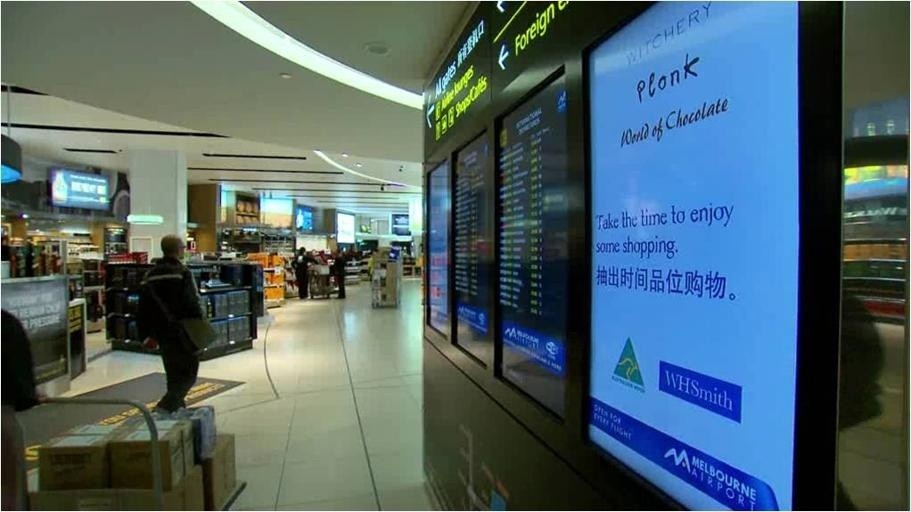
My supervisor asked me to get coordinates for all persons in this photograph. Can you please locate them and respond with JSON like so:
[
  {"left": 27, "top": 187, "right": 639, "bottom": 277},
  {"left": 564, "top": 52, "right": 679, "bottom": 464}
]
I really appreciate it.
[
  {"left": 138, "top": 235, "right": 207, "bottom": 414},
  {"left": 291, "top": 247, "right": 318, "bottom": 299},
  {"left": 315, "top": 249, "right": 347, "bottom": 299}
]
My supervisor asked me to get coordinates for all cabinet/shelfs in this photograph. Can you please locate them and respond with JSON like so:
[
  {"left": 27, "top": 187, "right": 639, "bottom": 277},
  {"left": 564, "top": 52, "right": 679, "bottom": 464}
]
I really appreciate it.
[{"left": 65, "top": 252, "right": 286, "bottom": 361}]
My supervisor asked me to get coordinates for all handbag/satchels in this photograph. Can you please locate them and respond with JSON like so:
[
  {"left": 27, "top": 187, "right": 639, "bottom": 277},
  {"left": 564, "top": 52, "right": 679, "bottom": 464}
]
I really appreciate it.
[{"left": 179, "top": 316, "right": 217, "bottom": 356}]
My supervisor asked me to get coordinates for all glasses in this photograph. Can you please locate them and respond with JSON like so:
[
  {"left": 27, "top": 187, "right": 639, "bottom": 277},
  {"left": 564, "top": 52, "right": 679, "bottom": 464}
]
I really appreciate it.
[{"left": 178, "top": 245, "right": 188, "bottom": 248}]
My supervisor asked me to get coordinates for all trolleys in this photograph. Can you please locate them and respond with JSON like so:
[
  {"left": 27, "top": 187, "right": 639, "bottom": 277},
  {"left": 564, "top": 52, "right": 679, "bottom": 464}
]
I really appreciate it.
[
  {"left": 307, "top": 265, "right": 341, "bottom": 299},
  {"left": 1, "top": 398, "right": 248, "bottom": 511}
]
